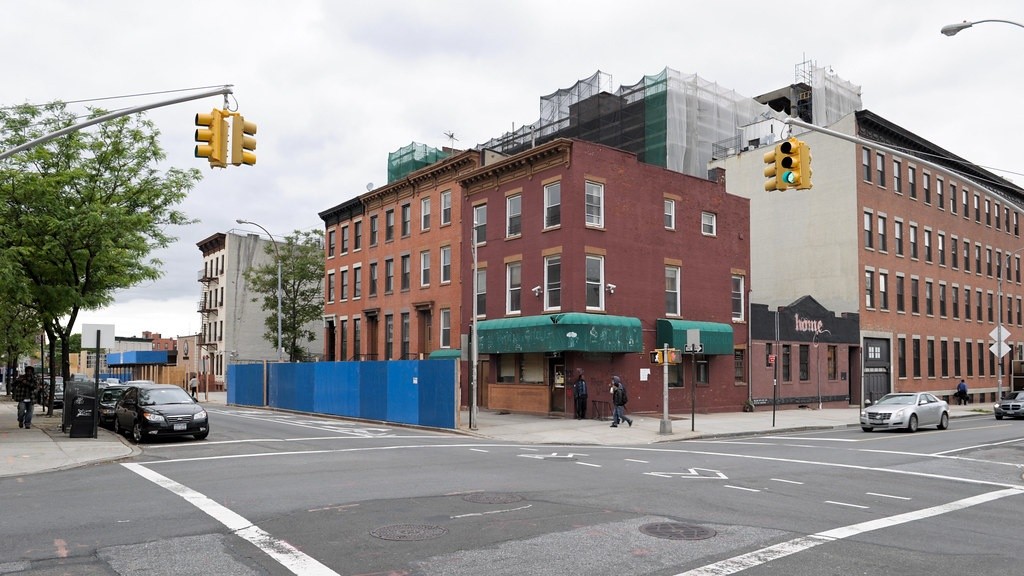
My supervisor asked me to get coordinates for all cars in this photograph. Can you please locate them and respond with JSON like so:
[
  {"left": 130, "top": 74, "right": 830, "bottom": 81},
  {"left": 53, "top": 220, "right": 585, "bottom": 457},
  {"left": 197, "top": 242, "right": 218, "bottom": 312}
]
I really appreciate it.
[
  {"left": 112, "top": 383, "right": 210, "bottom": 445},
  {"left": 8, "top": 372, "right": 156, "bottom": 427},
  {"left": 859, "top": 391, "right": 952, "bottom": 434},
  {"left": 992, "top": 390, "right": 1024, "bottom": 421}
]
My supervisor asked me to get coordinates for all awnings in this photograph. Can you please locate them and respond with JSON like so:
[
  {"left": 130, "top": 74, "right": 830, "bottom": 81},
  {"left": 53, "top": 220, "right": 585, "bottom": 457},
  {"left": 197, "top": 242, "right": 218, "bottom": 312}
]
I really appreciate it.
[
  {"left": 656, "top": 318, "right": 733, "bottom": 355},
  {"left": 429, "top": 349, "right": 462, "bottom": 359},
  {"left": 476, "top": 313, "right": 644, "bottom": 353}
]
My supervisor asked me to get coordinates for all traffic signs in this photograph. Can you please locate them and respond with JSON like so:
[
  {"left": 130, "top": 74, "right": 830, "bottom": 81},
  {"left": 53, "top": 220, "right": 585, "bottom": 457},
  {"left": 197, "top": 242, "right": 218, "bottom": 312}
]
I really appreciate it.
[{"left": 684, "top": 343, "right": 704, "bottom": 353}]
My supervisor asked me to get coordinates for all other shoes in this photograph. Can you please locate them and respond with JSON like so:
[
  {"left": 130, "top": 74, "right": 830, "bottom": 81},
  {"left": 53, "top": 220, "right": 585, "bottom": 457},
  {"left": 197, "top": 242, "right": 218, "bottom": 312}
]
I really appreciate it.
[
  {"left": 26, "top": 424, "right": 30, "bottom": 428},
  {"left": 19, "top": 422, "right": 23, "bottom": 427},
  {"left": 610, "top": 424, "right": 617, "bottom": 427},
  {"left": 629, "top": 420, "right": 633, "bottom": 427},
  {"left": 621, "top": 418, "right": 625, "bottom": 423}
]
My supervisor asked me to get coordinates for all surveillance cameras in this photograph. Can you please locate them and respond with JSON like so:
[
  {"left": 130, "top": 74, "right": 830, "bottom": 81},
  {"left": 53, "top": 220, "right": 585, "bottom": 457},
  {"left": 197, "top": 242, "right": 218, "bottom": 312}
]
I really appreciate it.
[
  {"left": 607, "top": 284, "right": 617, "bottom": 289},
  {"left": 532, "top": 286, "right": 542, "bottom": 293}
]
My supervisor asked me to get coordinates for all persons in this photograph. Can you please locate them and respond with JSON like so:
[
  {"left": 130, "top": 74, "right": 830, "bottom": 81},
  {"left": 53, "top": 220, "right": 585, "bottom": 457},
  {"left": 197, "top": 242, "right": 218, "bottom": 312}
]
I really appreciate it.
[
  {"left": 38, "top": 377, "right": 49, "bottom": 405},
  {"left": 189, "top": 376, "right": 200, "bottom": 396},
  {"left": 608, "top": 376, "right": 633, "bottom": 428},
  {"left": 574, "top": 374, "right": 588, "bottom": 420},
  {"left": 957, "top": 379, "right": 967, "bottom": 405},
  {"left": 10, "top": 366, "right": 39, "bottom": 429}
]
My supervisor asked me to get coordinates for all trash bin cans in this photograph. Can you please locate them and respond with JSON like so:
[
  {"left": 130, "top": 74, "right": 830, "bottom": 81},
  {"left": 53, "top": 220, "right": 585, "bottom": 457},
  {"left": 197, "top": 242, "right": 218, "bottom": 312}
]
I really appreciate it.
[
  {"left": 70, "top": 395, "right": 96, "bottom": 437},
  {"left": 63, "top": 381, "right": 95, "bottom": 426}
]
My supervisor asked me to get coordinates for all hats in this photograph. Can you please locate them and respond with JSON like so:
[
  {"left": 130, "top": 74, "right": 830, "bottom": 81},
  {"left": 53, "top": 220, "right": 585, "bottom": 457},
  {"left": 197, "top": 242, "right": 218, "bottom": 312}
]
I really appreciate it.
[{"left": 25, "top": 366, "right": 34, "bottom": 373}]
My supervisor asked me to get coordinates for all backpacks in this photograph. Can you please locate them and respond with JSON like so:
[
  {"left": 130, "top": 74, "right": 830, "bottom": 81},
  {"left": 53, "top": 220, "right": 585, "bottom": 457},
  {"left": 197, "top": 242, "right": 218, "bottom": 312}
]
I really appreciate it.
[{"left": 622, "top": 391, "right": 627, "bottom": 403}]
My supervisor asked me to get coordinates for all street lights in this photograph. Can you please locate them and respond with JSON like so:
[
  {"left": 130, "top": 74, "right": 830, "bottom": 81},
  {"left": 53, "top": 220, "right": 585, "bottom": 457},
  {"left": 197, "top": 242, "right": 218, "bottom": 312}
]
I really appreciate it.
[{"left": 236, "top": 218, "right": 282, "bottom": 362}]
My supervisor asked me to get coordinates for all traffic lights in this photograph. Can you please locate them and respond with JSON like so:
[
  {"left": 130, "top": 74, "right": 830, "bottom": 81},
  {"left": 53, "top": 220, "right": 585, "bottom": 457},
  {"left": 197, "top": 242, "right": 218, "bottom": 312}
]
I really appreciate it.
[
  {"left": 232, "top": 115, "right": 257, "bottom": 167},
  {"left": 195, "top": 108, "right": 223, "bottom": 163},
  {"left": 668, "top": 350, "right": 683, "bottom": 364},
  {"left": 763, "top": 146, "right": 787, "bottom": 192},
  {"left": 779, "top": 139, "right": 802, "bottom": 187},
  {"left": 649, "top": 351, "right": 663, "bottom": 364}
]
